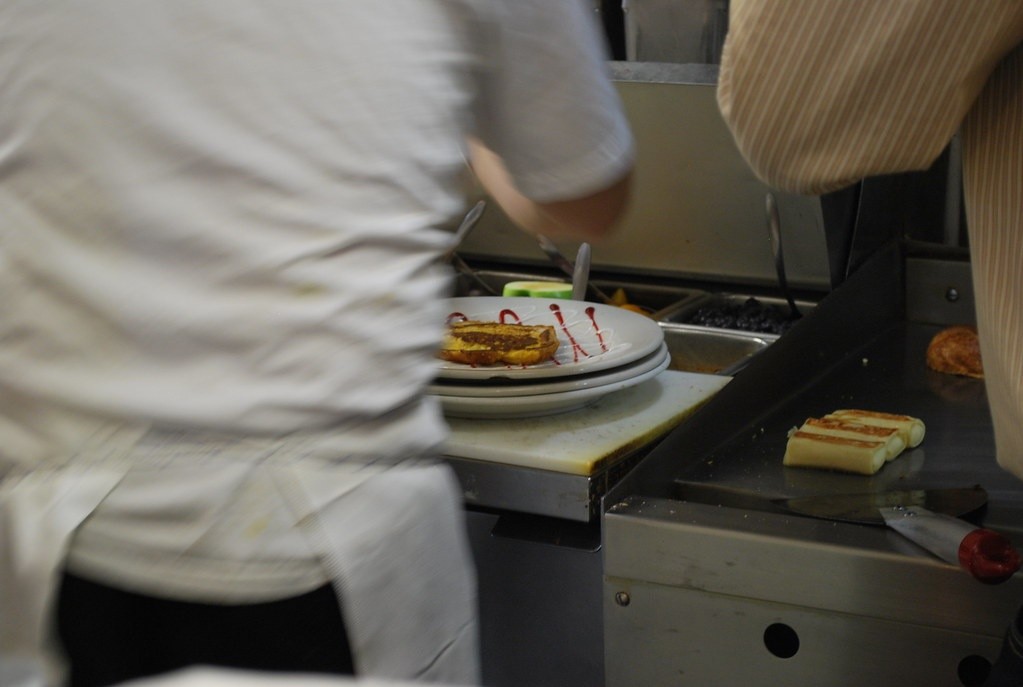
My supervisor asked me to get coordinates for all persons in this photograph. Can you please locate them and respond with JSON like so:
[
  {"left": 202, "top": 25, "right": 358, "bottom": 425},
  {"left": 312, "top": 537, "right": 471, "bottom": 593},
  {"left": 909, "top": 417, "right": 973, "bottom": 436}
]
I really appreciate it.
[
  {"left": 716, "top": 0, "right": 1023, "bottom": 687},
  {"left": 0, "top": 0, "right": 635, "bottom": 687}
]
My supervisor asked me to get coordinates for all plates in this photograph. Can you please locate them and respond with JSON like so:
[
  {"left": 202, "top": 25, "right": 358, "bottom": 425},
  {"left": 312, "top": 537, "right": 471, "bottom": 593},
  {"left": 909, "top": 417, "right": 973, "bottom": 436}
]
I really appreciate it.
[{"left": 426, "top": 297, "right": 671, "bottom": 419}]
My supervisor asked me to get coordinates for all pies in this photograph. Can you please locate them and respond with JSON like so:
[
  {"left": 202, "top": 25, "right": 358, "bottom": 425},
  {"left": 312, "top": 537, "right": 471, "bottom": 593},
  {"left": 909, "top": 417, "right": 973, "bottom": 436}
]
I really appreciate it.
[{"left": 783, "top": 408, "right": 926, "bottom": 475}]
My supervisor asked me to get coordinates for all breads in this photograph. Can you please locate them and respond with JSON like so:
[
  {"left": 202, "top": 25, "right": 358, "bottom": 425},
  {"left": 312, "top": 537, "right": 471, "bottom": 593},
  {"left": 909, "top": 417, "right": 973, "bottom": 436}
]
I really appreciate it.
[
  {"left": 434, "top": 320, "right": 560, "bottom": 364},
  {"left": 925, "top": 325, "right": 984, "bottom": 379}
]
